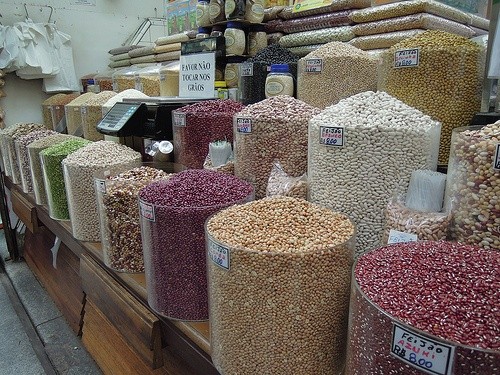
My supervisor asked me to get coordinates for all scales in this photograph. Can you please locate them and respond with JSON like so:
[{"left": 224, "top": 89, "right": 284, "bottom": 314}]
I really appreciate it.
[{"left": 96, "top": 89, "right": 220, "bottom": 145}]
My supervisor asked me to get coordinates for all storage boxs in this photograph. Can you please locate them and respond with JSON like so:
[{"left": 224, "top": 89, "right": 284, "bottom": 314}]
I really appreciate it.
[{"left": 2, "top": 47, "right": 499, "bottom": 375}]
